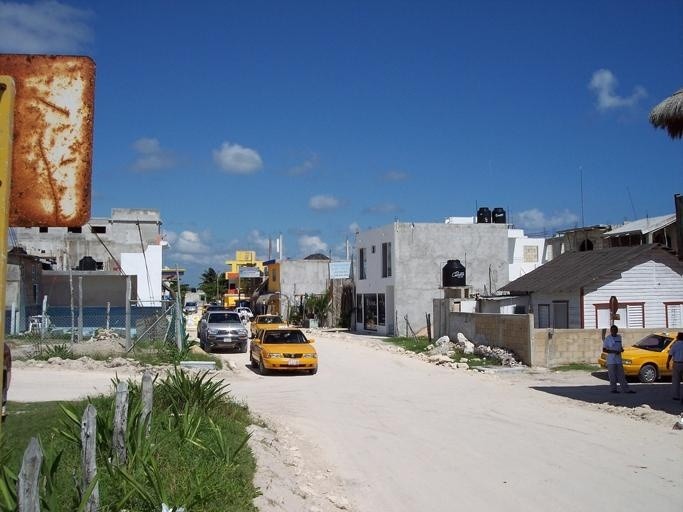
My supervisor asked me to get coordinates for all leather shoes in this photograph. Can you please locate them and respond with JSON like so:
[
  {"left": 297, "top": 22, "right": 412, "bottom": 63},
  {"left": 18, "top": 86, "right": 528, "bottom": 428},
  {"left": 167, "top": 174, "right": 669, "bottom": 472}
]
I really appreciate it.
[{"left": 611, "top": 390, "right": 636, "bottom": 393}]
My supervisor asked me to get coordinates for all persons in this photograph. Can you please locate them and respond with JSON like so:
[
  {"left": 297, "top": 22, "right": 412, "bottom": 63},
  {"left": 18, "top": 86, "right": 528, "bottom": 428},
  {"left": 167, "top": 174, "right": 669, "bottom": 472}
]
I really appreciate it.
[
  {"left": 601, "top": 325, "right": 636, "bottom": 395},
  {"left": 665, "top": 333, "right": 683, "bottom": 401}
]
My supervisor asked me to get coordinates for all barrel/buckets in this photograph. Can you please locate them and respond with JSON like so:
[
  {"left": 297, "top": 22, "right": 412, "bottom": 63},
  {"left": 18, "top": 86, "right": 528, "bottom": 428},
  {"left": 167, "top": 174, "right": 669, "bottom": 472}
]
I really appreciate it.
[
  {"left": 443, "top": 259, "right": 465, "bottom": 285},
  {"left": 477, "top": 207, "right": 491, "bottom": 223},
  {"left": 492, "top": 207, "right": 506, "bottom": 223}
]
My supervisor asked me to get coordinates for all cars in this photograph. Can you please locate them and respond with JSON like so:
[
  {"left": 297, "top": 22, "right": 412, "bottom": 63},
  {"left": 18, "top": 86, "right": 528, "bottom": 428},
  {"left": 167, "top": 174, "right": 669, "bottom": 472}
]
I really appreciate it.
[
  {"left": 250, "top": 326, "right": 318, "bottom": 376},
  {"left": 183, "top": 301, "right": 199, "bottom": 315},
  {"left": 598, "top": 331, "right": 683, "bottom": 383},
  {"left": 197, "top": 303, "right": 289, "bottom": 353}
]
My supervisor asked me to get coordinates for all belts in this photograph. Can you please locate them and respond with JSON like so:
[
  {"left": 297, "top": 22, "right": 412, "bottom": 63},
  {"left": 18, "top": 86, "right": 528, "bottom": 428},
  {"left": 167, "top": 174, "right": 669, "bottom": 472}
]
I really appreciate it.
[{"left": 674, "top": 361, "right": 683, "bottom": 363}]
